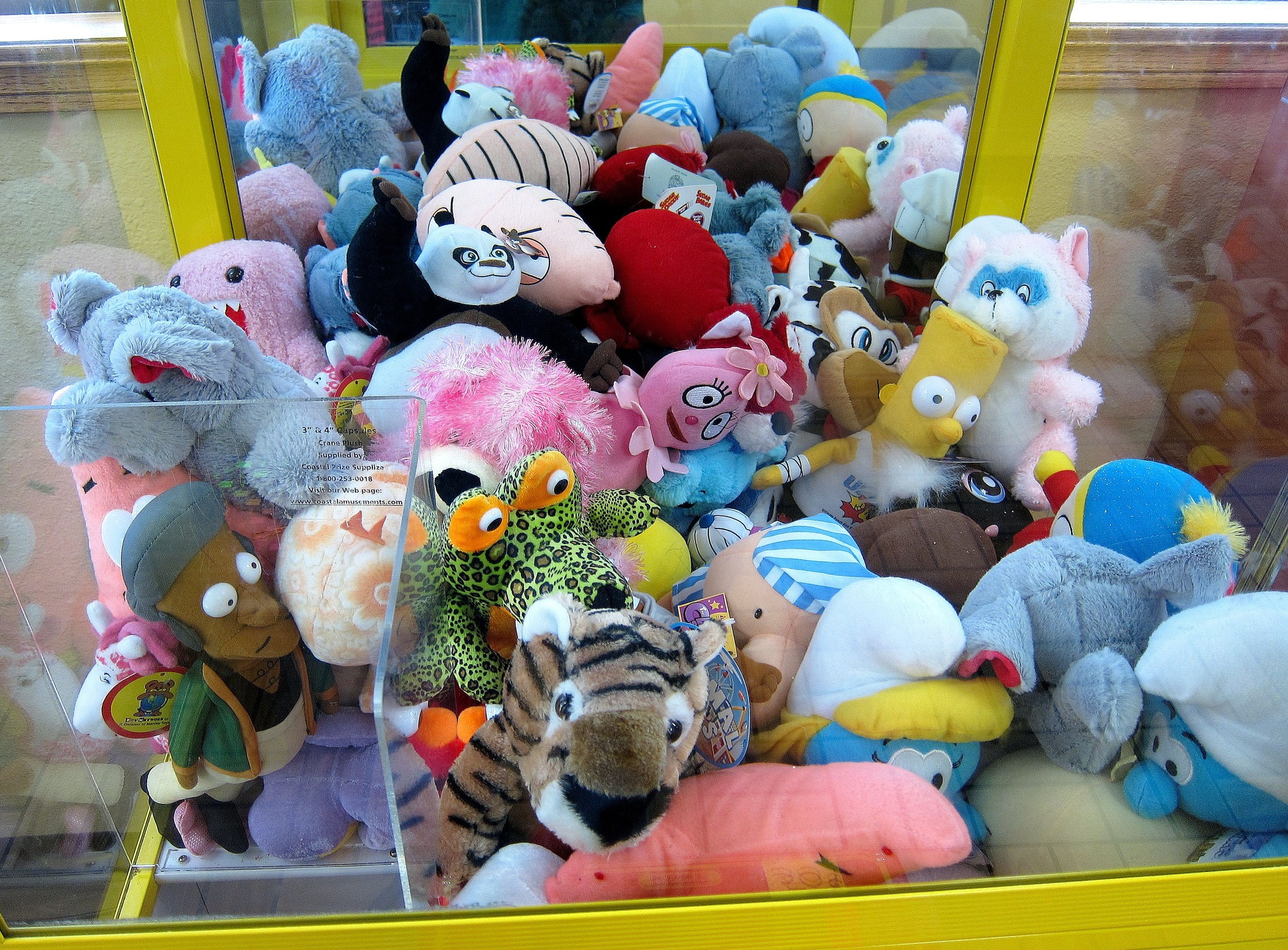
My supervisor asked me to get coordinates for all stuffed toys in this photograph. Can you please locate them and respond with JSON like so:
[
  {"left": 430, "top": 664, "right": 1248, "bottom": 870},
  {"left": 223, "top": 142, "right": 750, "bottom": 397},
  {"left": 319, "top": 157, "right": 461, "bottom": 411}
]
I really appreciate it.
[
  {"left": 0, "top": 380, "right": 164, "bottom": 773},
  {"left": 42, "top": 3, "right": 1288, "bottom": 912}
]
